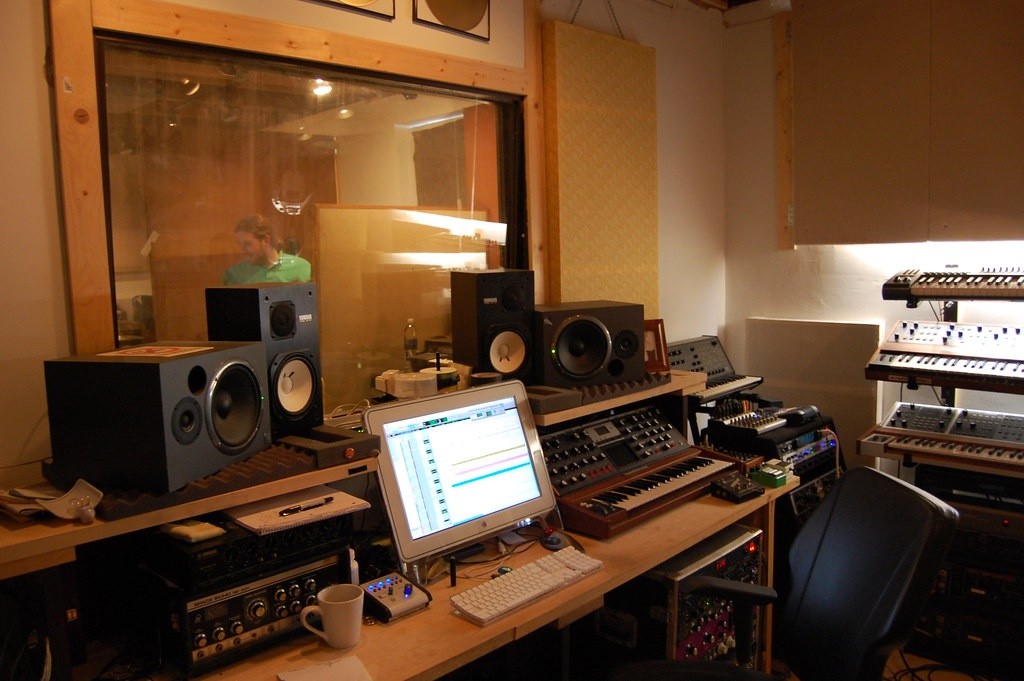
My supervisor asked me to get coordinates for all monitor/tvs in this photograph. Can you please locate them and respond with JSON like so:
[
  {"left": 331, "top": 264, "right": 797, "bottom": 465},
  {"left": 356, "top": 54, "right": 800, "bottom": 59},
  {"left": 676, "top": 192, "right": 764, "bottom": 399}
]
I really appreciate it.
[{"left": 360, "top": 378, "right": 564, "bottom": 588}]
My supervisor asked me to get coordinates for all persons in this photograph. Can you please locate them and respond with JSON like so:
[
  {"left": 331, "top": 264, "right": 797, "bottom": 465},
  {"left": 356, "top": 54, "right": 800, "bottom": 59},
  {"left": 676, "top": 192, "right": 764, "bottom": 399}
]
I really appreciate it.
[{"left": 221, "top": 215, "right": 311, "bottom": 283}]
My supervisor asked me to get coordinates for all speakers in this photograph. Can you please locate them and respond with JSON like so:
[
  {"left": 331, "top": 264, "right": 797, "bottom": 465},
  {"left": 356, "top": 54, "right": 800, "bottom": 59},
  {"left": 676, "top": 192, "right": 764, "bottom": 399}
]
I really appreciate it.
[
  {"left": 206, "top": 282, "right": 324, "bottom": 438},
  {"left": 45, "top": 340, "right": 273, "bottom": 495},
  {"left": 533, "top": 300, "right": 645, "bottom": 389},
  {"left": 450, "top": 270, "right": 536, "bottom": 386}
]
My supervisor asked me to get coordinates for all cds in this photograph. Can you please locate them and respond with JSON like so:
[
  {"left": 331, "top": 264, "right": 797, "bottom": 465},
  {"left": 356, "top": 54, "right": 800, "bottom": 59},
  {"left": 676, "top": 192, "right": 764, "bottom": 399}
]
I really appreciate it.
[{"left": 420, "top": 367, "right": 456, "bottom": 374}]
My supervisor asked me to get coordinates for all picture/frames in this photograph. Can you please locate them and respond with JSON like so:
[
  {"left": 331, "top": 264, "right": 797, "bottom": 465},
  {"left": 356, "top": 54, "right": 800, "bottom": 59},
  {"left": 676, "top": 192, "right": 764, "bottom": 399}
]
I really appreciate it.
[
  {"left": 412, "top": 0, "right": 491, "bottom": 41},
  {"left": 312, "top": 0, "right": 395, "bottom": 19}
]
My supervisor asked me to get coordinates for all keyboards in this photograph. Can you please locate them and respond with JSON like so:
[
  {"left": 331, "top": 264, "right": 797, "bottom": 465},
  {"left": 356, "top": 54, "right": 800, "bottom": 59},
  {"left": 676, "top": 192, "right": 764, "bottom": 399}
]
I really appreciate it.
[{"left": 450, "top": 546, "right": 604, "bottom": 628}]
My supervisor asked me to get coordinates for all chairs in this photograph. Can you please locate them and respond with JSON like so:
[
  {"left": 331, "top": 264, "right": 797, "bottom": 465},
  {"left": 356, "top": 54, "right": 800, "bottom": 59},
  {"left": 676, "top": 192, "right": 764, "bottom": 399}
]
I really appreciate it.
[{"left": 644, "top": 465, "right": 960, "bottom": 681}]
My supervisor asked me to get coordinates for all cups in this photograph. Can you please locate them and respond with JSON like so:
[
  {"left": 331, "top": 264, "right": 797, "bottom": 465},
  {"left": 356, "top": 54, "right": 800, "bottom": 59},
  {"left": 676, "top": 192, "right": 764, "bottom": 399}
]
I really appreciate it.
[{"left": 300, "top": 584, "right": 364, "bottom": 649}]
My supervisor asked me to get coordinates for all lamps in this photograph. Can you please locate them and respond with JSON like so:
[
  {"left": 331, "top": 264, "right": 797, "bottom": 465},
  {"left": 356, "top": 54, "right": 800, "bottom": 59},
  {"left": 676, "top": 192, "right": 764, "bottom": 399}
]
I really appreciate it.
[{"left": 161, "top": 59, "right": 417, "bottom": 141}]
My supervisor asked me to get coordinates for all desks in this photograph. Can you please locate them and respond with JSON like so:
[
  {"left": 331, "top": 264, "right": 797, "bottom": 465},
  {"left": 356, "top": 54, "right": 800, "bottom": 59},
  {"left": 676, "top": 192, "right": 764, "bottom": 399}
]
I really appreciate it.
[{"left": 191, "top": 475, "right": 800, "bottom": 681}]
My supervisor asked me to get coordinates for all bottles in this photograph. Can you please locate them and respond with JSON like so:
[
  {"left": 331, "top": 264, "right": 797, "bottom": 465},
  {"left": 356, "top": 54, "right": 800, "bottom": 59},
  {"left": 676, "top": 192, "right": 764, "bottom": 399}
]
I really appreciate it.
[
  {"left": 349, "top": 550, "right": 359, "bottom": 587},
  {"left": 404, "top": 319, "right": 417, "bottom": 358}
]
